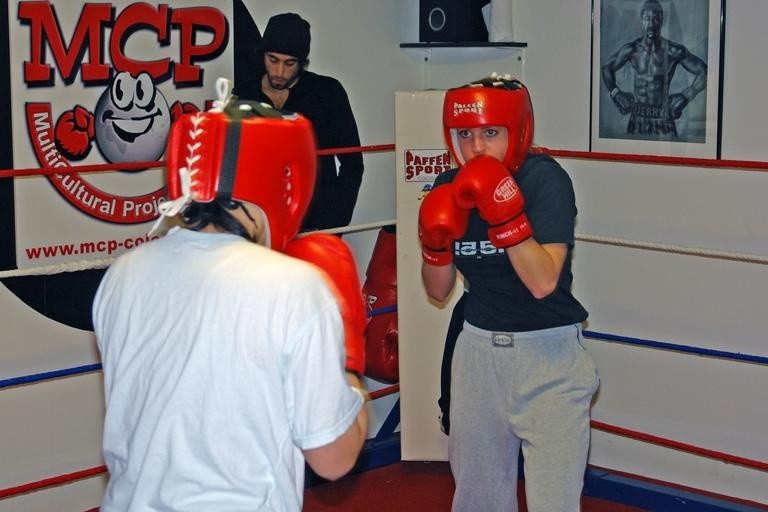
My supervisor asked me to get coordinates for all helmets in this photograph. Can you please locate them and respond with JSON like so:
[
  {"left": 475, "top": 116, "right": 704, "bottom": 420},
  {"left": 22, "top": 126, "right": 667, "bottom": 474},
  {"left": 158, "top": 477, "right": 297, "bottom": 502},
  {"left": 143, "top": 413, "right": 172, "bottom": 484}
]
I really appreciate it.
[
  {"left": 442, "top": 75, "right": 534, "bottom": 176},
  {"left": 164, "top": 96, "right": 318, "bottom": 254}
]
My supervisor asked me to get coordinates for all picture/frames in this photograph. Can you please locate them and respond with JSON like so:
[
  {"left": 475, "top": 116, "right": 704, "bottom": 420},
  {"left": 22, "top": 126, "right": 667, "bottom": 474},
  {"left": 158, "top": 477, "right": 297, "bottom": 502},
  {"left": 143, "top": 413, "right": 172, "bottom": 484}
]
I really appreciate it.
[{"left": 586, "top": 0, "right": 728, "bottom": 160}]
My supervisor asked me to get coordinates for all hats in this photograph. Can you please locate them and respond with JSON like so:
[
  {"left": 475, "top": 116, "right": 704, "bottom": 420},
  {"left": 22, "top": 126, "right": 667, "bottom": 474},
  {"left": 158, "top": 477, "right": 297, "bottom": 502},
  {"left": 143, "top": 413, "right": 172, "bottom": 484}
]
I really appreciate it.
[{"left": 259, "top": 12, "right": 311, "bottom": 61}]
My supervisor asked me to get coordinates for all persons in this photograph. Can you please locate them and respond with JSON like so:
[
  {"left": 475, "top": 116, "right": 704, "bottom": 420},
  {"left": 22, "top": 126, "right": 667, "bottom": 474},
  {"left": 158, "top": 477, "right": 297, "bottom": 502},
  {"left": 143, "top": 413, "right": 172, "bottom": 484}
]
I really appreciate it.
[
  {"left": 416, "top": 67, "right": 603, "bottom": 511},
  {"left": 601, "top": 1, "right": 708, "bottom": 145},
  {"left": 88, "top": 100, "right": 372, "bottom": 509},
  {"left": 234, "top": 12, "right": 367, "bottom": 253}
]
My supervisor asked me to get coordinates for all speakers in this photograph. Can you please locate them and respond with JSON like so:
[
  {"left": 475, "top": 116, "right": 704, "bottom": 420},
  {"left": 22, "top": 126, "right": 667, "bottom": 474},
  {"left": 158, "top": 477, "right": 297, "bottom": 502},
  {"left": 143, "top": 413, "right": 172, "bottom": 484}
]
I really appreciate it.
[{"left": 419, "top": 0, "right": 489, "bottom": 42}]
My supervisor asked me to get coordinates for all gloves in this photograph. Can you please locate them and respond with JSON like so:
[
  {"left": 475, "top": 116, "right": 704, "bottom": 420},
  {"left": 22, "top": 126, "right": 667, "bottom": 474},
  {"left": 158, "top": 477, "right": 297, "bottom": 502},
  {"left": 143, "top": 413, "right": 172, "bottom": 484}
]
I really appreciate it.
[
  {"left": 452, "top": 155, "right": 535, "bottom": 250},
  {"left": 284, "top": 230, "right": 370, "bottom": 379},
  {"left": 418, "top": 181, "right": 471, "bottom": 269},
  {"left": 609, "top": 88, "right": 635, "bottom": 114},
  {"left": 362, "top": 224, "right": 399, "bottom": 386},
  {"left": 669, "top": 88, "right": 697, "bottom": 120}
]
